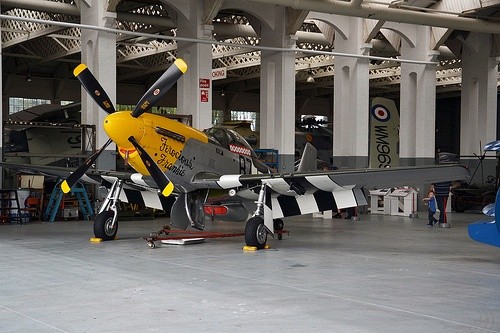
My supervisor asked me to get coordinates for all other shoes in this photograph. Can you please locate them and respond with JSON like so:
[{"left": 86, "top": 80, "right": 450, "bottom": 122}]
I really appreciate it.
[
  {"left": 434, "top": 220, "right": 438, "bottom": 225},
  {"left": 345, "top": 215, "right": 351, "bottom": 219},
  {"left": 427, "top": 224, "right": 433, "bottom": 226},
  {"left": 332, "top": 215, "right": 342, "bottom": 218}
]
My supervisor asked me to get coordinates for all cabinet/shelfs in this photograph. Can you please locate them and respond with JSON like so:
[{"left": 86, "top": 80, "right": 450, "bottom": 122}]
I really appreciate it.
[{"left": 44, "top": 198, "right": 79, "bottom": 221}]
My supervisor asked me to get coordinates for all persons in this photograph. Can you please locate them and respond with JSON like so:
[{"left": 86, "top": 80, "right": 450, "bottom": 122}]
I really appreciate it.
[
  {"left": 431, "top": 181, "right": 451, "bottom": 223},
  {"left": 422, "top": 190, "right": 438, "bottom": 226}
]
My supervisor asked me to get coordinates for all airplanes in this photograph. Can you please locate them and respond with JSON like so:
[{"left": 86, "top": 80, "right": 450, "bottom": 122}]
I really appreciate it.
[
  {"left": 0, "top": 58, "right": 473, "bottom": 251},
  {"left": 467, "top": 141, "right": 500, "bottom": 250}
]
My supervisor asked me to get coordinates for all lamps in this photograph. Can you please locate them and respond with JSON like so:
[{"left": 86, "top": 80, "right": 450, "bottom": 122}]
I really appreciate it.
[{"left": 306, "top": 69, "right": 314, "bottom": 82}]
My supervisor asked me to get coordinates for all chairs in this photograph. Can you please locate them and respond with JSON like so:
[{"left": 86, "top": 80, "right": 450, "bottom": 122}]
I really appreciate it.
[{"left": 17, "top": 197, "right": 40, "bottom": 224}]
